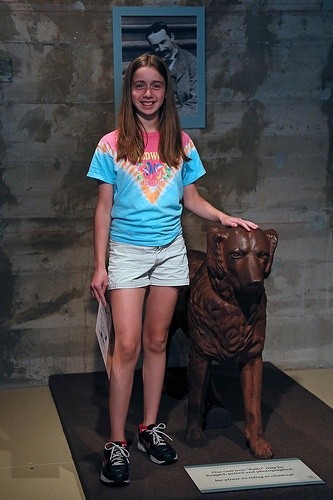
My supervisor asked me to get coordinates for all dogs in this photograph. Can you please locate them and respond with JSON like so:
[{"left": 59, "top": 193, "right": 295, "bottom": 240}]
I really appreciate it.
[{"left": 179, "top": 219, "right": 279, "bottom": 458}]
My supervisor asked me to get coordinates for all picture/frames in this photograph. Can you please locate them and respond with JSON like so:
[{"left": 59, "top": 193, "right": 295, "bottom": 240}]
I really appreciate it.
[{"left": 112, "top": 6, "right": 206, "bottom": 130}]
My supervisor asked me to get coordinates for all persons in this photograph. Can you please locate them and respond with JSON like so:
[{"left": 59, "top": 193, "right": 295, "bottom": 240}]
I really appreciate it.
[
  {"left": 86, "top": 52, "right": 260, "bottom": 486},
  {"left": 145, "top": 20, "right": 199, "bottom": 116}
]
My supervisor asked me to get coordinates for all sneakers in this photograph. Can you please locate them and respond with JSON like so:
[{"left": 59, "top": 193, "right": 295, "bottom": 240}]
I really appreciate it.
[
  {"left": 100, "top": 440, "right": 132, "bottom": 485},
  {"left": 137, "top": 421, "right": 178, "bottom": 466}
]
